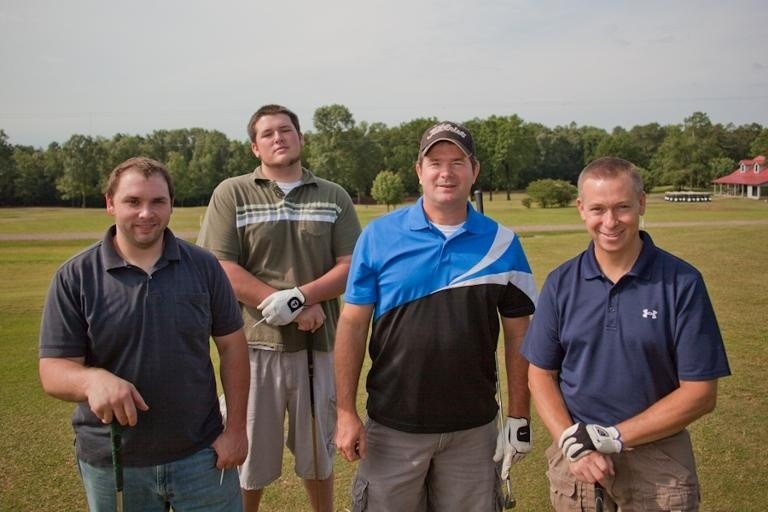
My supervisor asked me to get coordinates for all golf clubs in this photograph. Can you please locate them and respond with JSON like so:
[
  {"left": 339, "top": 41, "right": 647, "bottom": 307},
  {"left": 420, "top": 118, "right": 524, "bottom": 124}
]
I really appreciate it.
[{"left": 475, "top": 192, "right": 515, "bottom": 510}]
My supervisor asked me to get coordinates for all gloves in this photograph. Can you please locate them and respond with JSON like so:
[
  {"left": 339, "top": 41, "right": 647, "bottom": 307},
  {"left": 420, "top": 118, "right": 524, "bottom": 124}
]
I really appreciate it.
[
  {"left": 494, "top": 416, "right": 532, "bottom": 480},
  {"left": 557, "top": 421, "right": 623, "bottom": 463},
  {"left": 256, "top": 286, "right": 305, "bottom": 328}
]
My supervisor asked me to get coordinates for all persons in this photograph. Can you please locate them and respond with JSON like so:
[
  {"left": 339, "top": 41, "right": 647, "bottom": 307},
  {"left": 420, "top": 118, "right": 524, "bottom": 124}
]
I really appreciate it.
[
  {"left": 333, "top": 120, "right": 540, "bottom": 512},
  {"left": 518, "top": 156, "right": 733, "bottom": 512},
  {"left": 195, "top": 104, "right": 362, "bottom": 512},
  {"left": 38, "top": 156, "right": 253, "bottom": 512}
]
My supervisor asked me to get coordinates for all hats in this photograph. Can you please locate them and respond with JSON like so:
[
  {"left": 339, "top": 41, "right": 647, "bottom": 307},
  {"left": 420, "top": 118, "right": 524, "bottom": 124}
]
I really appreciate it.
[{"left": 421, "top": 122, "right": 474, "bottom": 157}]
